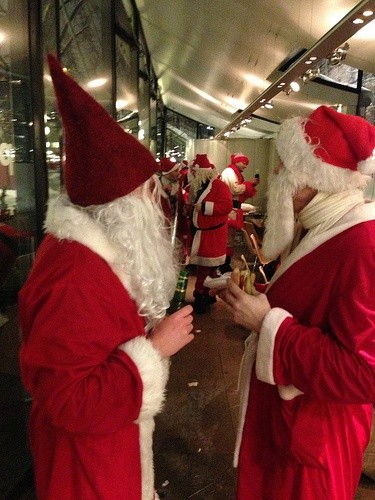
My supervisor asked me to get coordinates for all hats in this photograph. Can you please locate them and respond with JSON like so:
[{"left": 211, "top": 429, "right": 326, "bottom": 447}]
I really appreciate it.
[
  {"left": 45, "top": 52, "right": 159, "bottom": 207},
  {"left": 276, "top": 105, "right": 375, "bottom": 193},
  {"left": 158, "top": 158, "right": 180, "bottom": 175},
  {"left": 181, "top": 159, "right": 188, "bottom": 167},
  {"left": 230, "top": 152, "right": 249, "bottom": 164},
  {"left": 191, "top": 154, "right": 215, "bottom": 170}
]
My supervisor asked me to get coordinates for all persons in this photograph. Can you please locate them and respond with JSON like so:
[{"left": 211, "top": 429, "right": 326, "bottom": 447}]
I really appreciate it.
[
  {"left": 210, "top": 127, "right": 374, "bottom": 499},
  {"left": 186, "top": 156, "right": 233, "bottom": 315},
  {"left": 156, "top": 156, "right": 191, "bottom": 260},
  {"left": 17, "top": 144, "right": 193, "bottom": 500},
  {"left": 178, "top": 160, "right": 189, "bottom": 191},
  {"left": 219, "top": 151, "right": 260, "bottom": 274}
]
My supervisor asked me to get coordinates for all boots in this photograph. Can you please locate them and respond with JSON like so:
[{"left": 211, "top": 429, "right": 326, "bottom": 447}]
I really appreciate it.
[
  {"left": 219, "top": 255, "right": 234, "bottom": 274},
  {"left": 192, "top": 286, "right": 218, "bottom": 312}
]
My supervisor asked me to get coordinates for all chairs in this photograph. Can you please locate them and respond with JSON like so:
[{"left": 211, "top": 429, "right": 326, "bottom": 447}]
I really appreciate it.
[{"left": 242, "top": 219, "right": 285, "bottom": 275}]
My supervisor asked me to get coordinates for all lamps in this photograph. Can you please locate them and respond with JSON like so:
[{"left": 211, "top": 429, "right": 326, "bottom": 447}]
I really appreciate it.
[
  {"left": 290, "top": 77, "right": 305, "bottom": 92},
  {"left": 221, "top": 118, "right": 253, "bottom": 137},
  {"left": 330, "top": 43, "right": 351, "bottom": 64},
  {"left": 263, "top": 99, "right": 275, "bottom": 110}
]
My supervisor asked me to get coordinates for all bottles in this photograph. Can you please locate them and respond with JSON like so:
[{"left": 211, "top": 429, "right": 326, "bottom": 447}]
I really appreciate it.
[{"left": 166, "top": 270, "right": 190, "bottom": 316}]
